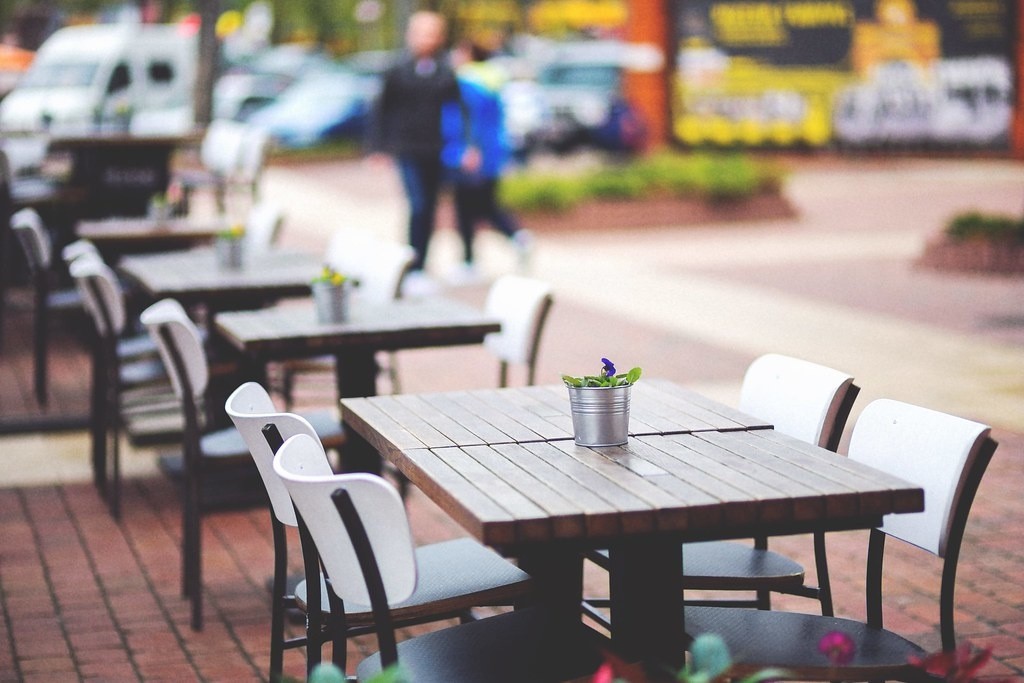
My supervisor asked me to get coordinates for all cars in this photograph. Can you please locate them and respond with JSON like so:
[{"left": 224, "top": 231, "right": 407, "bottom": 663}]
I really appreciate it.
[{"left": 203, "top": 0, "right": 1024, "bottom": 161}]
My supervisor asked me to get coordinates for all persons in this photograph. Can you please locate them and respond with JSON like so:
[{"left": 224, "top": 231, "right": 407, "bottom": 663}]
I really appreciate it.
[
  {"left": 374, "top": 12, "right": 480, "bottom": 265},
  {"left": 439, "top": 41, "right": 535, "bottom": 273}
]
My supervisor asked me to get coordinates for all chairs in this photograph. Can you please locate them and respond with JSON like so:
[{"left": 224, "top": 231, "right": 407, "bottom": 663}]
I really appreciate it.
[
  {"left": 473, "top": 276, "right": 550, "bottom": 388},
  {"left": 0, "top": 146, "right": 245, "bottom": 519},
  {"left": 227, "top": 382, "right": 533, "bottom": 683},
  {"left": 274, "top": 433, "right": 607, "bottom": 683},
  {"left": 680, "top": 398, "right": 997, "bottom": 682},
  {"left": 580, "top": 352, "right": 860, "bottom": 632},
  {"left": 139, "top": 297, "right": 348, "bottom": 634},
  {"left": 201, "top": 118, "right": 414, "bottom": 395}
]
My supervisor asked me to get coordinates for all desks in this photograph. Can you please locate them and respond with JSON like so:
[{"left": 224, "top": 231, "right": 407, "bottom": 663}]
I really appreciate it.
[
  {"left": 74, "top": 216, "right": 234, "bottom": 251},
  {"left": 340, "top": 376, "right": 925, "bottom": 617},
  {"left": 119, "top": 245, "right": 372, "bottom": 421},
  {"left": 213, "top": 293, "right": 501, "bottom": 473}
]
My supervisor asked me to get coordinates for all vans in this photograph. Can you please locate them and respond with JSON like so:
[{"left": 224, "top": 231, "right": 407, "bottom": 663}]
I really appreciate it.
[{"left": 0, "top": 22, "right": 202, "bottom": 146}]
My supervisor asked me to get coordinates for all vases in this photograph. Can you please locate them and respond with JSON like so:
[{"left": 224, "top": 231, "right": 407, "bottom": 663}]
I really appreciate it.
[
  {"left": 567, "top": 384, "right": 632, "bottom": 447},
  {"left": 313, "top": 283, "right": 351, "bottom": 322}
]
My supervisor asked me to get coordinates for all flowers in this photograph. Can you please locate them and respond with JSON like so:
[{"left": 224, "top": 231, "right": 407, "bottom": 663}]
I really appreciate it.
[
  {"left": 219, "top": 226, "right": 245, "bottom": 239},
  {"left": 588, "top": 629, "right": 992, "bottom": 683},
  {"left": 151, "top": 184, "right": 184, "bottom": 211},
  {"left": 564, "top": 358, "right": 642, "bottom": 388},
  {"left": 310, "top": 267, "right": 349, "bottom": 286}
]
